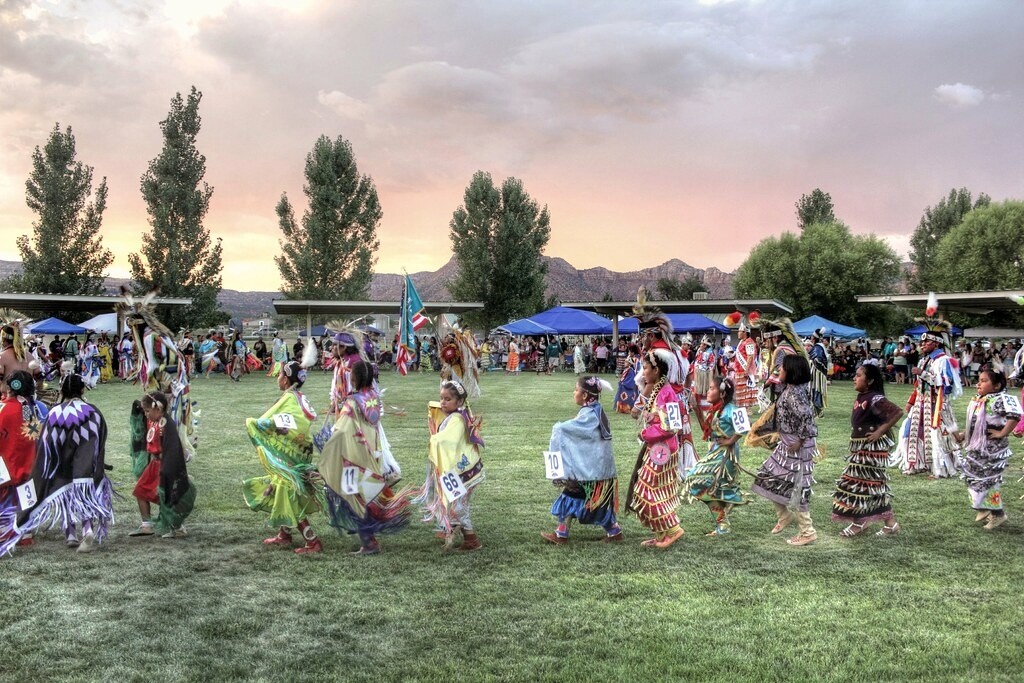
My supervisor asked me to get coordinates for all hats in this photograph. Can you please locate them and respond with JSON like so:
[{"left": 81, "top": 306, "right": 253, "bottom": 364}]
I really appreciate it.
[
  {"left": 993, "top": 349, "right": 1001, "bottom": 354},
  {"left": 960, "top": 337, "right": 966, "bottom": 343}
]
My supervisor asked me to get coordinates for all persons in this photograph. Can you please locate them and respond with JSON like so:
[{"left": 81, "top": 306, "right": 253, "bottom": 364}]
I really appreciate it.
[
  {"left": 0, "top": 284, "right": 304, "bottom": 558},
  {"left": 441, "top": 333, "right": 464, "bottom": 386},
  {"left": 734, "top": 322, "right": 759, "bottom": 416},
  {"left": 313, "top": 331, "right": 1024, "bottom": 414},
  {"left": 750, "top": 318, "right": 827, "bottom": 420},
  {"left": 541, "top": 376, "right": 623, "bottom": 546},
  {"left": 315, "top": 360, "right": 402, "bottom": 556},
  {"left": 241, "top": 361, "right": 323, "bottom": 553},
  {"left": 889, "top": 331, "right": 967, "bottom": 479},
  {"left": 624, "top": 356, "right": 903, "bottom": 548},
  {"left": 631, "top": 284, "right": 700, "bottom": 482},
  {"left": 313, "top": 318, "right": 401, "bottom": 488},
  {"left": 808, "top": 328, "right": 826, "bottom": 418},
  {"left": 411, "top": 381, "right": 484, "bottom": 551},
  {"left": 953, "top": 368, "right": 1021, "bottom": 529}
]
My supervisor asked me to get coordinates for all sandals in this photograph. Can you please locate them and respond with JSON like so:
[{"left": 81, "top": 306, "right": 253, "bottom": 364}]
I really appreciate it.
[
  {"left": 873, "top": 523, "right": 900, "bottom": 538},
  {"left": 839, "top": 522, "right": 869, "bottom": 538}
]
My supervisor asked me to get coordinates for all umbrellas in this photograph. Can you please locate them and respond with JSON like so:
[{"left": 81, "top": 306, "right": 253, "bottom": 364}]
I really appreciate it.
[{"left": 905, "top": 325, "right": 959, "bottom": 335}]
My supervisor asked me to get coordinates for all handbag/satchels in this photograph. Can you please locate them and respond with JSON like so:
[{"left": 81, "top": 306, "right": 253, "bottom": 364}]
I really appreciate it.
[{"left": 827, "top": 362, "right": 834, "bottom": 377}]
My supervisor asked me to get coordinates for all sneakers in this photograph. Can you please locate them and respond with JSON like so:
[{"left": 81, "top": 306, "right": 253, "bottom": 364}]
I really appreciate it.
[
  {"left": 601, "top": 534, "right": 623, "bottom": 542},
  {"left": 349, "top": 543, "right": 381, "bottom": 557},
  {"left": 540, "top": 530, "right": 567, "bottom": 545}
]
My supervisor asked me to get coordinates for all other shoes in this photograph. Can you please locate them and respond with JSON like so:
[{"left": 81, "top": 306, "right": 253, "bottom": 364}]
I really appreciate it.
[
  {"left": 812, "top": 404, "right": 824, "bottom": 420},
  {"left": 655, "top": 529, "right": 685, "bottom": 548},
  {"left": 66, "top": 540, "right": 81, "bottom": 546},
  {"left": 76, "top": 532, "right": 93, "bottom": 553},
  {"left": 706, "top": 531, "right": 718, "bottom": 537},
  {"left": 161, "top": 529, "right": 187, "bottom": 538},
  {"left": 640, "top": 538, "right": 658, "bottom": 547},
  {"left": 15, "top": 538, "right": 35, "bottom": 549},
  {"left": 983, "top": 515, "right": 1008, "bottom": 531},
  {"left": 974, "top": 510, "right": 990, "bottom": 522},
  {"left": 770, "top": 510, "right": 796, "bottom": 534},
  {"left": 128, "top": 527, "right": 155, "bottom": 535},
  {"left": 86, "top": 383, "right": 92, "bottom": 391},
  {"left": 787, "top": 533, "right": 818, "bottom": 545}
]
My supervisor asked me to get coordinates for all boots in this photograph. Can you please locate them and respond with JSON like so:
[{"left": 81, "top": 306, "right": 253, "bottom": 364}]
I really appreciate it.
[
  {"left": 262, "top": 525, "right": 293, "bottom": 546},
  {"left": 293, "top": 520, "right": 323, "bottom": 554},
  {"left": 452, "top": 534, "right": 483, "bottom": 551}
]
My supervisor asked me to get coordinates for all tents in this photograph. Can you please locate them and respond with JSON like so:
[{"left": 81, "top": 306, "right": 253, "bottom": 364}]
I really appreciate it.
[
  {"left": 21, "top": 316, "right": 88, "bottom": 345},
  {"left": 77, "top": 312, "right": 132, "bottom": 334},
  {"left": 792, "top": 314, "right": 866, "bottom": 339},
  {"left": 493, "top": 307, "right": 729, "bottom": 334}
]
